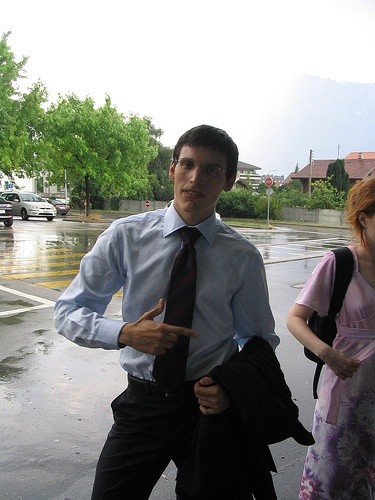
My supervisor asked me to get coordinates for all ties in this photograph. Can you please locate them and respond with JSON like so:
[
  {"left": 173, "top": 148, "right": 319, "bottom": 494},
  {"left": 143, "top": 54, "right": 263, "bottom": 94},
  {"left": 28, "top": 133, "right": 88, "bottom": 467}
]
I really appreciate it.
[{"left": 152, "top": 228, "right": 202, "bottom": 393}]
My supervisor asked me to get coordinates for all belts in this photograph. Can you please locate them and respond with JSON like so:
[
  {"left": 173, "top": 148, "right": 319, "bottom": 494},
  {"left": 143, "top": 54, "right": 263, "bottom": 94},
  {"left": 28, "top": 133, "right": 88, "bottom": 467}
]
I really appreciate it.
[{"left": 128, "top": 374, "right": 195, "bottom": 398}]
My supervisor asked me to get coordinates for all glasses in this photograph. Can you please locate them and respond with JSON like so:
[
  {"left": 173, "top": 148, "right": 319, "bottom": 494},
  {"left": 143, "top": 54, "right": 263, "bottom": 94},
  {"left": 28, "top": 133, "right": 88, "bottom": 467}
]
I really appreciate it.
[{"left": 173, "top": 159, "right": 228, "bottom": 177}]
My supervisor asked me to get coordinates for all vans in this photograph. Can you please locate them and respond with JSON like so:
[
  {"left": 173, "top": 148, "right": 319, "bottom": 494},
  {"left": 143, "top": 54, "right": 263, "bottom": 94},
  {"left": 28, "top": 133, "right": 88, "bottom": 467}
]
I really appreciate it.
[{"left": 0, "top": 192, "right": 57, "bottom": 222}]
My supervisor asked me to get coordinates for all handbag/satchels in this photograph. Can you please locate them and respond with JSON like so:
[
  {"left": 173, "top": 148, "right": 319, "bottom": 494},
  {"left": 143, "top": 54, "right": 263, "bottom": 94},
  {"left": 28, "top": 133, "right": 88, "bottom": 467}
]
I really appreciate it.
[{"left": 304, "top": 246, "right": 356, "bottom": 364}]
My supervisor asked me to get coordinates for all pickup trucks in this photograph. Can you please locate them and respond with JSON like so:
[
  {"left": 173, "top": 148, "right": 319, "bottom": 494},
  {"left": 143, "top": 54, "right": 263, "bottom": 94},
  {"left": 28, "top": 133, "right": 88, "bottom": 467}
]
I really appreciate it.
[{"left": 0, "top": 196, "right": 13, "bottom": 228}]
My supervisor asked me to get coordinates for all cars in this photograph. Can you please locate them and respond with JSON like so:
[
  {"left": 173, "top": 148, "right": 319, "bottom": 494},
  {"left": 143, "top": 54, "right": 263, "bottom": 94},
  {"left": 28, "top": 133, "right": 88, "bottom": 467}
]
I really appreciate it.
[{"left": 45, "top": 197, "right": 71, "bottom": 216}]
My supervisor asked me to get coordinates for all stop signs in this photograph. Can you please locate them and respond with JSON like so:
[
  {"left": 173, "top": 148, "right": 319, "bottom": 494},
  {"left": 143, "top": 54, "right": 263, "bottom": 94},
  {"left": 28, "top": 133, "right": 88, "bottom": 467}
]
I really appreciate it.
[
  {"left": 145, "top": 201, "right": 150, "bottom": 208},
  {"left": 266, "top": 177, "right": 273, "bottom": 187}
]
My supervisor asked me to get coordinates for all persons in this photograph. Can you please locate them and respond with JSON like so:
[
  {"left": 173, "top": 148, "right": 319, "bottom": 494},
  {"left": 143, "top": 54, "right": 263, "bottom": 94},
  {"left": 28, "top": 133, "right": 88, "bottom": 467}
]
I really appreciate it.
[
  {"left": 285, "top": 175, "right": 375, "bottom": 500},
  {"left": 53, "top": 125, "right": 280, "bottom": 500}
]
